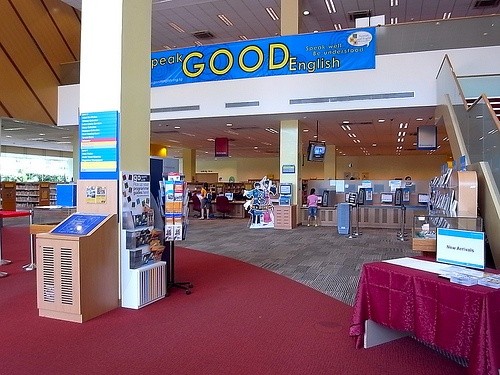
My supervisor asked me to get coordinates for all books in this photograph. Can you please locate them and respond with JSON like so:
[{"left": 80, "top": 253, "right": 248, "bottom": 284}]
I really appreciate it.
[
  {"left": 165, "top": 172, "right": 189, "bottom": 241},
  {"left": 429, "top": 169, "right": 457, "bottom": 229},
  {"left": 450, "top": 270, "right": 500, "bottom": 289}
]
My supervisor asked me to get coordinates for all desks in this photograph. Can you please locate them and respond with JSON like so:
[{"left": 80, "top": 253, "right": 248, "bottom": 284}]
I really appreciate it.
[{"left": 348, "top": 256, "right": 500, "bottom": 375}]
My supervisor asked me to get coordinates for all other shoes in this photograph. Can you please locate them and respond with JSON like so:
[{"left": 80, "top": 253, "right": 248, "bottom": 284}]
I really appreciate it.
[
  {"left": 207, "top": 217, "right": 210, "bottom": 220},
  {"left": 315, "top": 224, "right": 317, "bottom": 227},
  {"left": 199, "top": 217, "right": 204, "bottom": 220},
  {"left": 308, "top": 224, "right": 310, "bottom": 227}
]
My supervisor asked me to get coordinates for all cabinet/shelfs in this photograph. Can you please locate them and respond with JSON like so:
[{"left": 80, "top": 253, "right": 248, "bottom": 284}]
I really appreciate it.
[
  {"left": 0, "top": 181, "right": 57, "bottom": 211},
  {"left": 187, "top": 182, "right": 246, "bottom": 201}
]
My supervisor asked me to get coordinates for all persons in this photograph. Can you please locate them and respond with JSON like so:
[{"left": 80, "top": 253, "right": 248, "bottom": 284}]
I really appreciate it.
[
  {"left": 307, "top": 188, "right": 318, "bottom": 227},
  {"left": 198, "top": 183, "right": 210, "bottom": 220},
  {"left": 405, "top": 176, "right": 415, "bottom": 193}
]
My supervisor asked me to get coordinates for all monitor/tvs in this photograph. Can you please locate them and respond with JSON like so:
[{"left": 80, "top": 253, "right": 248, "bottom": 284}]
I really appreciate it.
[
  {"left": 418, "top": 194, "right": 429, "bottom": 203},
  {"left": 314, "top": 147, "right": 326, "bottom": 158},
  {"left": 317, "top": 197, "right": 322, "bottom": 203},
  {"left": 381, "top": 194, "right": 393, "bottom": 202},
  {"left": 225, "top": 193, "right": 233, "bottom": 201}
]
